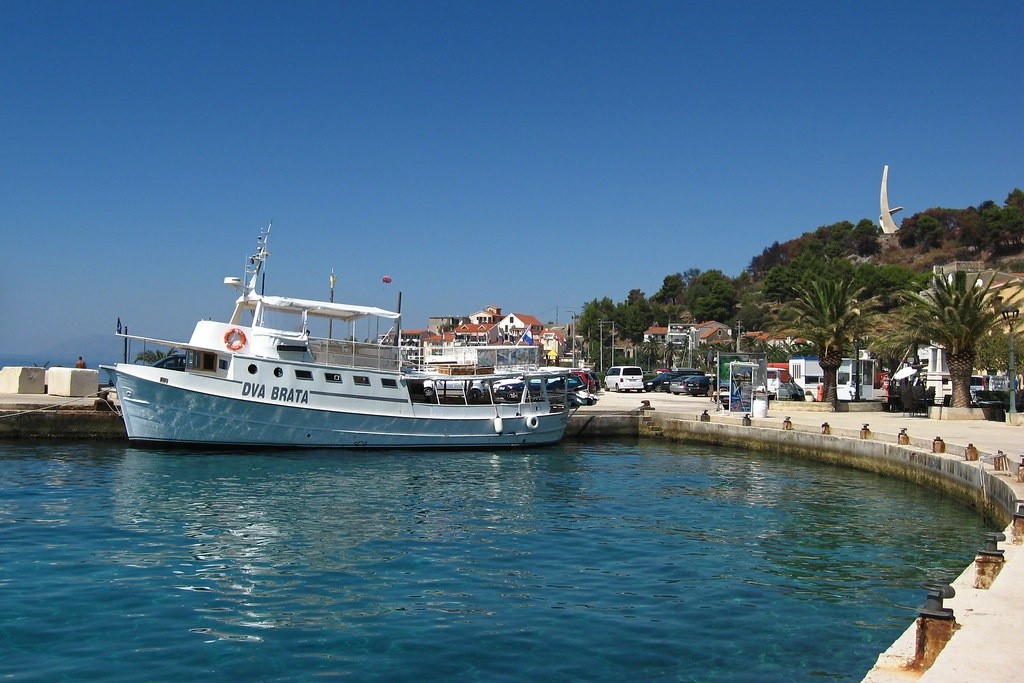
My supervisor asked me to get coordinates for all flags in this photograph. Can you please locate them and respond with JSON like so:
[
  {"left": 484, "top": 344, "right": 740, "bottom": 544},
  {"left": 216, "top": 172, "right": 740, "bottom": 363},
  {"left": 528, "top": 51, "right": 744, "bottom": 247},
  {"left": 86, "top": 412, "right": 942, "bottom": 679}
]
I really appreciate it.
[{"left": 523, "top": 327, "right": 534, "bottom": 346}]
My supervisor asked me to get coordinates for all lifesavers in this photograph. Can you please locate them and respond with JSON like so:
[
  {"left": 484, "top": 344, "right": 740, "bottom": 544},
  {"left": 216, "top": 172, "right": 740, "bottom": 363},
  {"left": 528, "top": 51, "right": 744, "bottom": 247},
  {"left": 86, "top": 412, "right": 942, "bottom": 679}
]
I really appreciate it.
[
  {"left": 527, "top": 416, "right": 539, "bottom": 429},
  {"left": 225, "top": 329, "right": 246, "bottom": 350}
]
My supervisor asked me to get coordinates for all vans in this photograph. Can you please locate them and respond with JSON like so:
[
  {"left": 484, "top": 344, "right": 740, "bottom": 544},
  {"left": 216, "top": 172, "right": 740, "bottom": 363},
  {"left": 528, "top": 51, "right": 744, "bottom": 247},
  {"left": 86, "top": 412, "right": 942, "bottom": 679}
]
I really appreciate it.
[
  {"left": 767, "top": 367, "right": 805, "bottom": 401},
  {"left": 603, "top": 365, "right": 644, "bottom": 393}
]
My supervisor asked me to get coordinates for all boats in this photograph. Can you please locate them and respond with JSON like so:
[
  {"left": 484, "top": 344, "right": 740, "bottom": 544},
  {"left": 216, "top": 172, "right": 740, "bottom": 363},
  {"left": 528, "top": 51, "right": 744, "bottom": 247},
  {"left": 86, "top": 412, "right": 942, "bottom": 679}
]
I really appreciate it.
[{"left": 97, "top": 215, "right": 584, "bottom": 451}]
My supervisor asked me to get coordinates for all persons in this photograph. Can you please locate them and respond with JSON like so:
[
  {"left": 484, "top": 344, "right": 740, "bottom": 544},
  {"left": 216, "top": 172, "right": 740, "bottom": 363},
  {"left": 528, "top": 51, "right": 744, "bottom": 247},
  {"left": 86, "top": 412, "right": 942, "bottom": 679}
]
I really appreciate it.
[
  {"left": 76, "top": 356, "right": 87, "bottom": 368},
  {"left": 1014, "top": 369, "right": 1023, "bottom": 394}
]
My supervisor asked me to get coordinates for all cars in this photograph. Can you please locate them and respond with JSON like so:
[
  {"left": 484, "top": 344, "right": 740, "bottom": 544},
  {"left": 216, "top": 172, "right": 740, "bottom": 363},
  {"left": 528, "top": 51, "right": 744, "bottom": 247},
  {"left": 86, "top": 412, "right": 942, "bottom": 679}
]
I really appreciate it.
[
  {"left": 421, "top": 365, "right": 601, "bottom": 407},
  {"left": 643, "top": 367, "right": 730, "bottom": 397},
  {"left": 147, "top": 351, "right": 194, "bottom": 371}
]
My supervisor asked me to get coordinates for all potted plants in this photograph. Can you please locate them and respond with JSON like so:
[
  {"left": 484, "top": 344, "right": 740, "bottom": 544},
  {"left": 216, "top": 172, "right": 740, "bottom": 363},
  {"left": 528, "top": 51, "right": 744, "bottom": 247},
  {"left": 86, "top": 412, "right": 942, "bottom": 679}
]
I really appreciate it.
[{"left": 805, "top": 391, "right": 814, "bottom": 402}]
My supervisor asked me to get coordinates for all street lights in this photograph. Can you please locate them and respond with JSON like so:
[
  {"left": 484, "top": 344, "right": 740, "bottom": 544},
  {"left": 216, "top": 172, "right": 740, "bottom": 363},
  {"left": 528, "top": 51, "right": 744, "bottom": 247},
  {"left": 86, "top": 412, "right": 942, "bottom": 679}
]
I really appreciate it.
[{"left": 1001, "top": 306, "right": 1019, "bottom": 413}]
